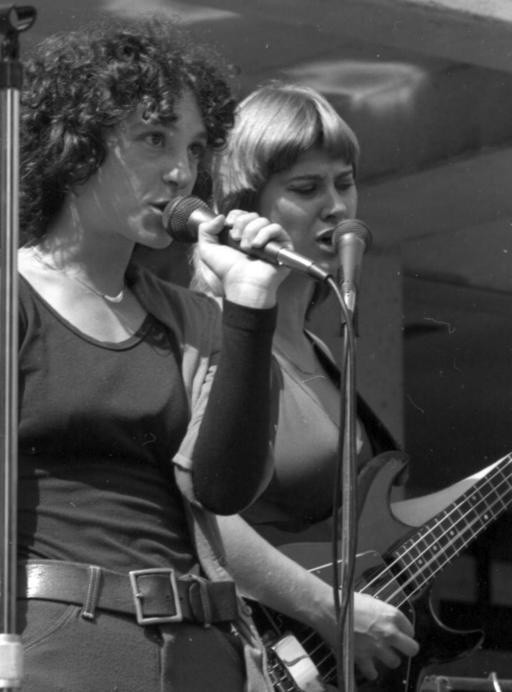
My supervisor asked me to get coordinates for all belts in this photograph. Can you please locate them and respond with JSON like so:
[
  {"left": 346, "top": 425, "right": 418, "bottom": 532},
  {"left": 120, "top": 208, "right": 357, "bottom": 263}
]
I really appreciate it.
[{"left": 16, "top": 559, "right": 240, "bottom": 627}]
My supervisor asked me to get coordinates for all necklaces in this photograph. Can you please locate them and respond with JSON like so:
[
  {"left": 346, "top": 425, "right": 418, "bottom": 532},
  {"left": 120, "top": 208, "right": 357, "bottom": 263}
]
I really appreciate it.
[
  {"left": 30, "top": 239, "right": 136, "bottom": 307},
  {"left": 271, "top": 339, "right": 327, "bottom": 380}
]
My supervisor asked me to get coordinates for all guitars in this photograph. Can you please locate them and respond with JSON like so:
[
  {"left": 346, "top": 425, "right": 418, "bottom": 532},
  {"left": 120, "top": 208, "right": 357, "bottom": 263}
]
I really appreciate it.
[{"left": 240, "top": 450, "right": 512, "bottom": 691}]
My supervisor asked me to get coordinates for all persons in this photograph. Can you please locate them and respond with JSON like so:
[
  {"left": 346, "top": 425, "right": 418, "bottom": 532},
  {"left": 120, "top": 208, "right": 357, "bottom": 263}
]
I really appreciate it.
[
  {"left": 185, "top": 80, "right": 427, "bottom": 692},
  {"left": 2, "top": 9, "right": 296, "bottom": 692}
]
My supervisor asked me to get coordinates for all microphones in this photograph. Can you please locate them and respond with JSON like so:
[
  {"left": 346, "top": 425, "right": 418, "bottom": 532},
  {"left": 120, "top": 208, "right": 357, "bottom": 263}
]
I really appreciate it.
[
  {"left": 161, "top": 195, "right": 333, "bottom": 283},
  {"left": 334, "top": 220, "right": 372, "bottom": 335}
]
